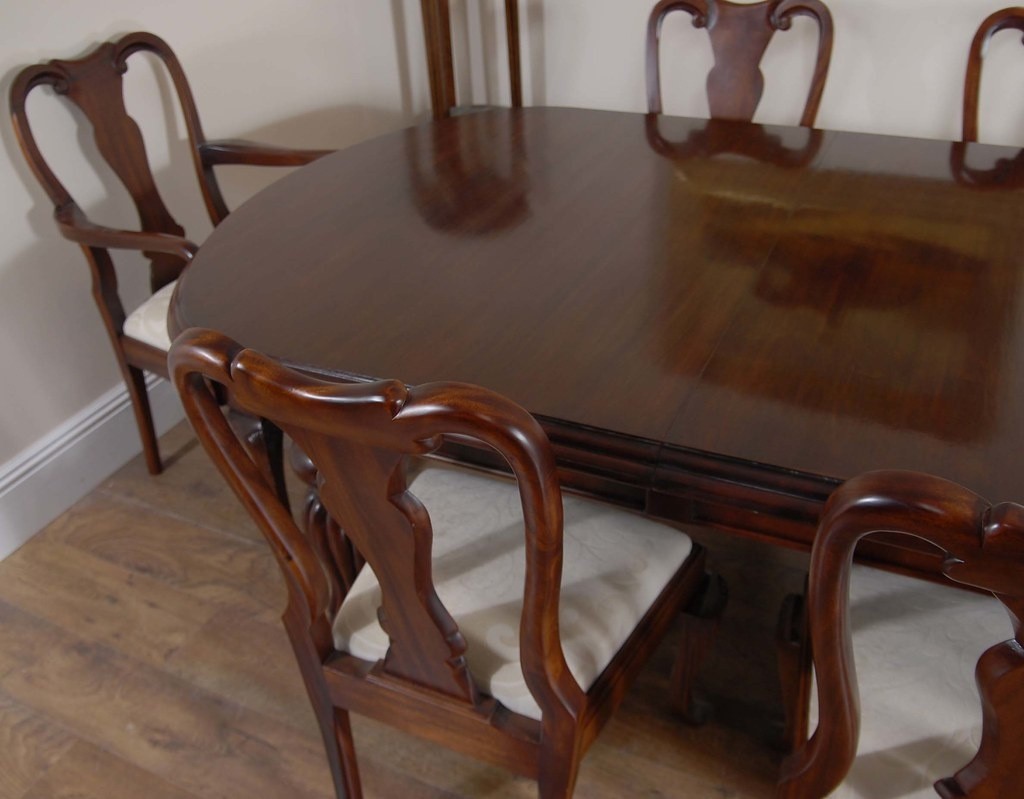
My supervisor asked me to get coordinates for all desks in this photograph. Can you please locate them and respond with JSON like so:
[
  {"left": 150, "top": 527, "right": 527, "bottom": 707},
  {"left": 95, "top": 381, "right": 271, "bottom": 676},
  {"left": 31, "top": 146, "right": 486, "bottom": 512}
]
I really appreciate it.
[{"left": 166, "top": 104, "right": 1024, "bottom": 595}]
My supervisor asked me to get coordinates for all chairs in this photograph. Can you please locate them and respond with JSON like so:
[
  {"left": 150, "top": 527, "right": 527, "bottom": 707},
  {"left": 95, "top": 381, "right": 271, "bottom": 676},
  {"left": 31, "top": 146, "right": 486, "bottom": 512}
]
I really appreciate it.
[
  {"left": 773, "top": 466, "right": 1024, "bottom": 799},
  {"left": 963, "top": 7, "right": 1024, "bottom": 143},
  {"left": 644, "top": 1, "right": 834, "bottom": 127},
  {"left": 8, "top": 31, "right": 335, "bottom": 517},
  {"left": 167, "top": 327, "right": 712, "bottom": 799}
]
